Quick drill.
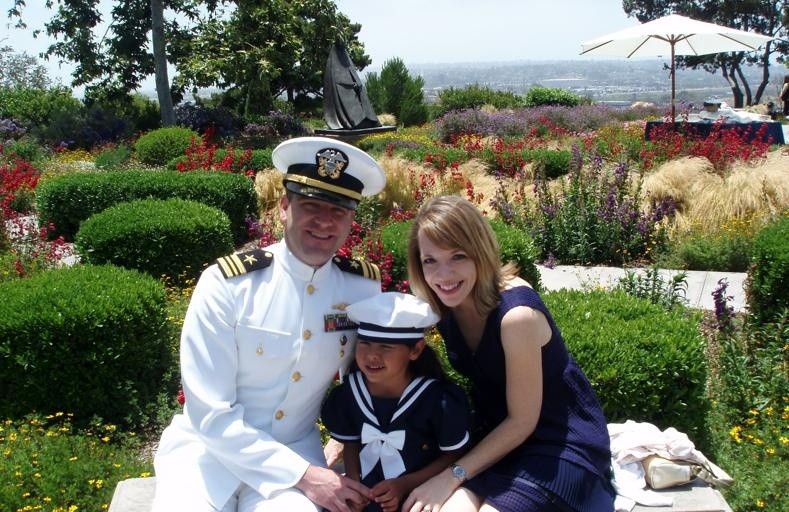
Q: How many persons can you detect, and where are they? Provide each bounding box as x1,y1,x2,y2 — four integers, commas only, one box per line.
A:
401,195,612,511
780,76,789,120
144,135,386,512
320,291,471,512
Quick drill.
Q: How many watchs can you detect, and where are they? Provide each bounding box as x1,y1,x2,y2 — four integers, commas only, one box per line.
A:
449,463,468,484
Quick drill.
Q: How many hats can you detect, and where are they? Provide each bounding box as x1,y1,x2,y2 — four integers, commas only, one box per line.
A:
344,292,440,339
271,137,386,211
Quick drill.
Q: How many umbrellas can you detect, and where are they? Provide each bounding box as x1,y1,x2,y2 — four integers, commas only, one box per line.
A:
577,13,776,132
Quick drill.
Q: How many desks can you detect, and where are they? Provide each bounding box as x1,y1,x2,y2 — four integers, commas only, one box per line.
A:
645,121,786,145
